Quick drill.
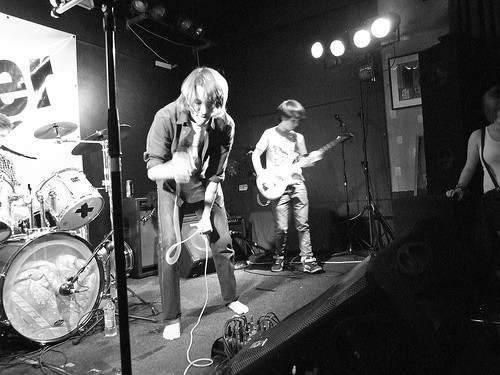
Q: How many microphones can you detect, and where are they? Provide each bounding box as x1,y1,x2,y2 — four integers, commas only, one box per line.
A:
50,0,82,19
334,114,346,128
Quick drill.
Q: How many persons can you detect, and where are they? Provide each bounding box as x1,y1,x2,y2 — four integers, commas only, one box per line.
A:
146,67,249,342
252,99,324,274
0,113,16,180
446,86,500,325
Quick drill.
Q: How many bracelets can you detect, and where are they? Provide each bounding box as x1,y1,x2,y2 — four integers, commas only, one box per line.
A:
455,185,466,191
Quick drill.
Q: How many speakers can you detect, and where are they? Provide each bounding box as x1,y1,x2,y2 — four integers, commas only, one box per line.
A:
392,198,477,312
178,220,216,279
419,38,490,196
122,197,158,278
376,214,482,325
222,253,442,375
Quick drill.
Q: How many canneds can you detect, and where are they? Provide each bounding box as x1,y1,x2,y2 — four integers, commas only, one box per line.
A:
126,180,133,197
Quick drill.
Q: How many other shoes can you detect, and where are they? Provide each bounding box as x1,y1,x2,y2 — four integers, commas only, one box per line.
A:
303,262,323,274
271,259,284,271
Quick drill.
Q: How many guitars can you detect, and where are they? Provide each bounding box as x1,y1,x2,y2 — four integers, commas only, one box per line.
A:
255,132,355,200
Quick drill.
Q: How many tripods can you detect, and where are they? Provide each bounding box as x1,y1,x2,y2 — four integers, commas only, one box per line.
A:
57,138,159,344
331,81,395,257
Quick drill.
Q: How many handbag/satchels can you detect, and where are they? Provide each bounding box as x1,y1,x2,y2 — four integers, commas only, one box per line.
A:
481,187,500,233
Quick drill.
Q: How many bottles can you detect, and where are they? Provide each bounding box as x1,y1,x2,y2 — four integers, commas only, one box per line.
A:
126,178,134,198
104,294,117,337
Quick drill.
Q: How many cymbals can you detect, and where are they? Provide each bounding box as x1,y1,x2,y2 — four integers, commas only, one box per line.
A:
33,121,77,140
71,124,132,156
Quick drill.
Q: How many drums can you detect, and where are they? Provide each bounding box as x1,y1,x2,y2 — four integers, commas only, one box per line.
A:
0,173,18,244
0,230,104,343
37,165,105,231
